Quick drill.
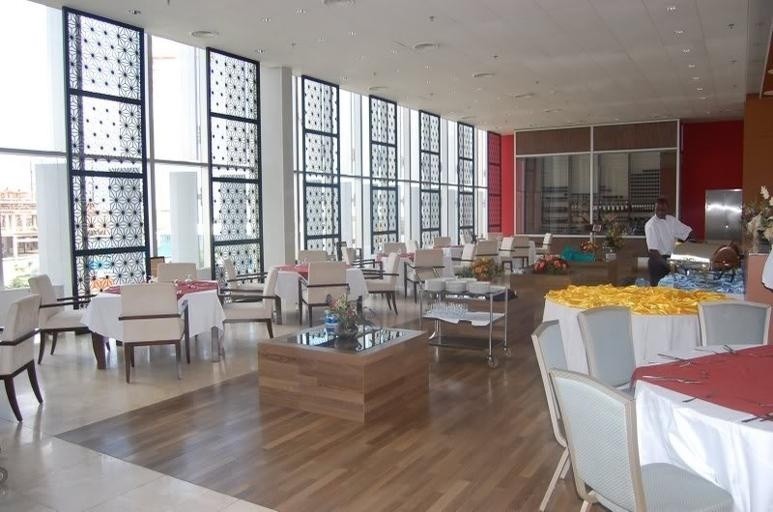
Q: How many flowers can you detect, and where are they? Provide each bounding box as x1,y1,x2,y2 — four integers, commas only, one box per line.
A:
603,226,626,252
739,187,771,253
471,256,498,280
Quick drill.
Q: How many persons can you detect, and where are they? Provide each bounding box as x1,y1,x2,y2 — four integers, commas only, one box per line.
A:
645,198,693,283
534,252,548,269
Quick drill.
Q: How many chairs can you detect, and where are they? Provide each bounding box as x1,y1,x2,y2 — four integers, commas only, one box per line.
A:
499,238,514,273
476,241,498,255
698,302,771,347
453,244,474,275
341,246,375,270
119,283,191,383
363,254,398,315
531,319,572,511
404,248,445,303
298,260,349,326
1,294,43,421
218,269,274,354
298,250,326,263
157,263,196,283
576,306,636,393
514,237,529,270
434,236,450,247
225,259,263,294
536,233,551,255
550,366,734,511
28,275,110,365
384,241,407,257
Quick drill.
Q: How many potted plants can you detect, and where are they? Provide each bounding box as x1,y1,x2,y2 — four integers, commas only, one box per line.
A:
323,293,378,337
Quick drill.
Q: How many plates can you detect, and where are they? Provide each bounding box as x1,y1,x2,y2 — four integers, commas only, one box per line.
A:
489,285,506,293
424,278,445,291
445,280,466,294
436,276,457,291
456,278,477,292
468,281,491,295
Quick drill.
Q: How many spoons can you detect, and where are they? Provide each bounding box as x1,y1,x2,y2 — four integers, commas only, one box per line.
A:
740,411,773,423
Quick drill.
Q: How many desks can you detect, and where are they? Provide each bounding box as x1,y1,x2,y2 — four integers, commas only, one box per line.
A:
277,266,369,326
544,284,730,377
632,342,772,512
80,281,226,369
